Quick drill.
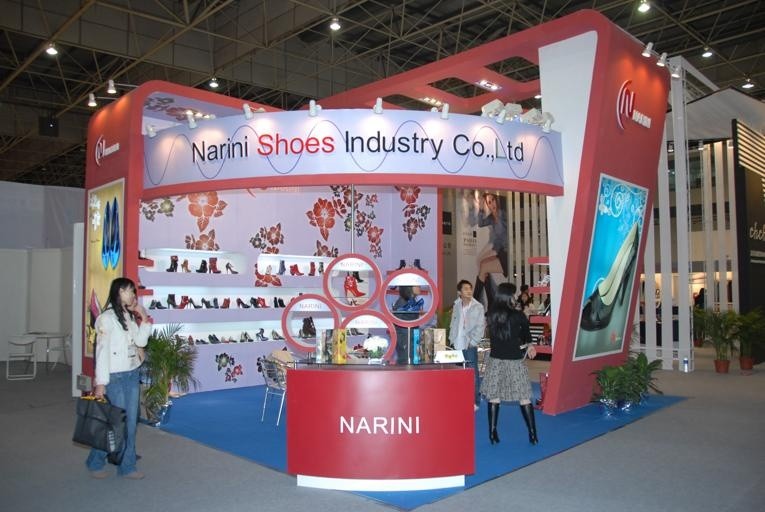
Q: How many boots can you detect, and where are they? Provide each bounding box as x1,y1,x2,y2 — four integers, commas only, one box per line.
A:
303,317,316,337
488,402,499,444
473,274,494,316
166,256,239,273
277,260,323,275
520,403,538,445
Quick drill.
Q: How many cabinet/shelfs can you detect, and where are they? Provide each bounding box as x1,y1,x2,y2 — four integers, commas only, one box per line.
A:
527,256,552,355
136,248,430,394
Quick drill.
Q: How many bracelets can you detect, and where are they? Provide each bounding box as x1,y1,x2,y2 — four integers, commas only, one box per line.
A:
93,391,104,396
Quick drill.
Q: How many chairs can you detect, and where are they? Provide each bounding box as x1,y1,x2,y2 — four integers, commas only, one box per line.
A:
259,358,293,427
5,337,37,381
50,333,73,372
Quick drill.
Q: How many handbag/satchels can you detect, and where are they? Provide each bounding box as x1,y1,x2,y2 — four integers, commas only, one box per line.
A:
72,394,128,454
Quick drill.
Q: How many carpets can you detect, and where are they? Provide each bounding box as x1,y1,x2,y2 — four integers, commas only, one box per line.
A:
157,374,690,510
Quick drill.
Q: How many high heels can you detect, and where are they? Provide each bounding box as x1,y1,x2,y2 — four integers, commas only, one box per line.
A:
102,198,120,270
188,329,283,344
397,259,423,270
149,294,285,309
581,223,638,331
344,272,365,296
396,297,424,311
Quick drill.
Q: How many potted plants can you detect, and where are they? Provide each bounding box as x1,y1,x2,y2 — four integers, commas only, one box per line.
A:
693,306,764,374
588,350,664,417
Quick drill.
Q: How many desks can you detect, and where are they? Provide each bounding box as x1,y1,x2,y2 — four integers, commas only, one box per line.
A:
24,332,70,378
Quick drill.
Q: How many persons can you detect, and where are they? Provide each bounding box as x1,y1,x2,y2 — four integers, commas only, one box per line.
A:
520,292,529,309
106,283,155,464
518,284,532,304
695,287,708,310
447,280,487,413
468,191,508,301
478,282,540,445
80,276,151,483
